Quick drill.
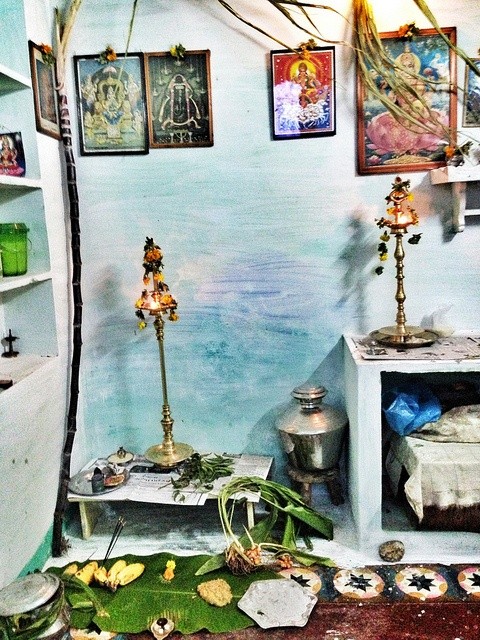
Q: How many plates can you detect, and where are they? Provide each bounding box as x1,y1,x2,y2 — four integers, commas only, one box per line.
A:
104,475,124,486
237,579,318,629
85,472,93,480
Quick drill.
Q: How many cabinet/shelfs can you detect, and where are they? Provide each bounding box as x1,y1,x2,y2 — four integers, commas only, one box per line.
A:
1,0,72,596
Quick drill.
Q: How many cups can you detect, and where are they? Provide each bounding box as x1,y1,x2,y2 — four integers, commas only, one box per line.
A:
92,474,103,493
0,222,33,277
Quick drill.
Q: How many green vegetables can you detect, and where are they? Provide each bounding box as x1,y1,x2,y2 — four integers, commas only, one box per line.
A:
170,452,334,572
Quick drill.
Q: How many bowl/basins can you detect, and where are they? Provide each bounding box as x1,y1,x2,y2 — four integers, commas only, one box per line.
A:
106,451,134,467
0,573,64,640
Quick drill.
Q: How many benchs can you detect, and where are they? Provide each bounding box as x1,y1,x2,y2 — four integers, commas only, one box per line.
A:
67,456,273,539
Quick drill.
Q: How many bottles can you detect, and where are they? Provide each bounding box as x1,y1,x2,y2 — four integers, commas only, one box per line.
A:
274,384,347,472
117,447,126,458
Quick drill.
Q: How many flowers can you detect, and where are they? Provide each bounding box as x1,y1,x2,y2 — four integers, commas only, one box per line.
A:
300,38,318,58
40,44,57,66
169,43,186,58
96,45,116,63
397,20,420,41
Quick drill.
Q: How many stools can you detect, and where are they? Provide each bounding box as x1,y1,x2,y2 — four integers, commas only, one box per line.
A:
285,465,344,506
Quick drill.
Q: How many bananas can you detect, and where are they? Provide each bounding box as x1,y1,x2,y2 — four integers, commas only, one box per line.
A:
64,561,145,592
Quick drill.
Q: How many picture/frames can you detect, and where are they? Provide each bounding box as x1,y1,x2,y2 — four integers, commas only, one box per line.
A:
28,40,63,141
270,46,336,140
73,51,149,156
144,50,213,148
461,58,480,128
355,27,457,176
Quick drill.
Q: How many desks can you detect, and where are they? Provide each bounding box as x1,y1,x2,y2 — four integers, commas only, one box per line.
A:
341,334,480,554
